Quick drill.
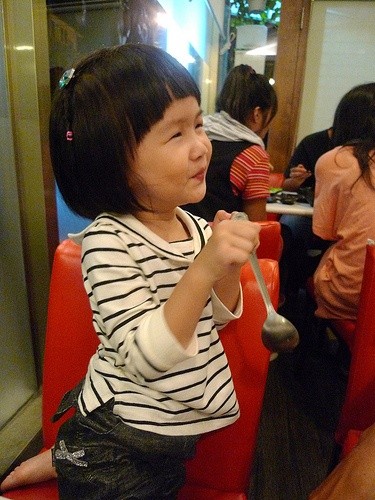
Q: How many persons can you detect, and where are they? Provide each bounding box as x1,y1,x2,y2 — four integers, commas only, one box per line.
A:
278,83,375,378
181,65,300,309
0,43,260,500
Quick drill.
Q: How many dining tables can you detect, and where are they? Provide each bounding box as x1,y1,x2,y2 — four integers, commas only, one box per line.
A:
266,200,314,215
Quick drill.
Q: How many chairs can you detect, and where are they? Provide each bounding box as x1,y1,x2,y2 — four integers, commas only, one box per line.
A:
307,238,375,445
207,221,284,263
1,239,279,500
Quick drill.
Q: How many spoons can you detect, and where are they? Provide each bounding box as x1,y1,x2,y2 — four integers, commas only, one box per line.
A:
231,212,299,354
